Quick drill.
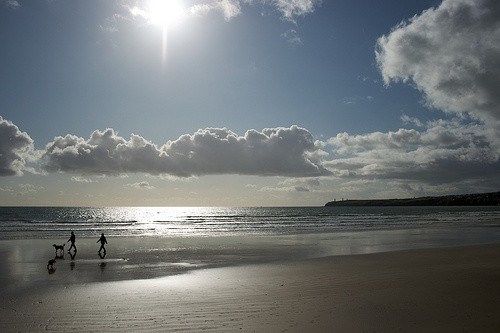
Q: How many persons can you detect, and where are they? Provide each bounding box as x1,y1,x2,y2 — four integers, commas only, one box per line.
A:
66,231,78,252
95,233,108,253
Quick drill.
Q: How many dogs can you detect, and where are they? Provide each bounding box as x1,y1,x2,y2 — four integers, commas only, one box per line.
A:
47,259,56,265
52,243,65,252
